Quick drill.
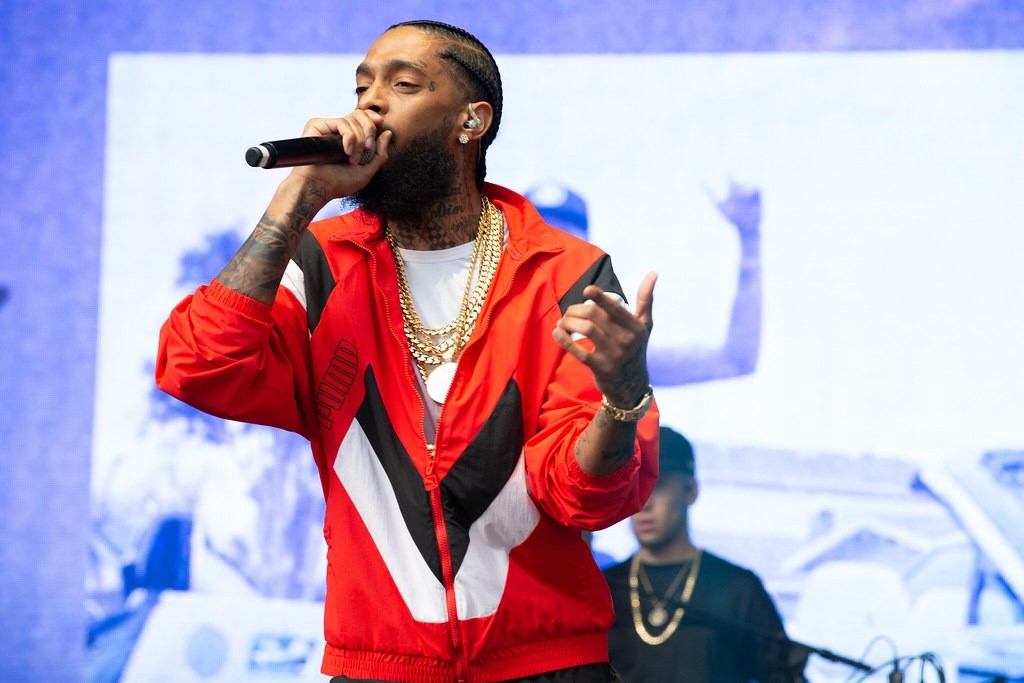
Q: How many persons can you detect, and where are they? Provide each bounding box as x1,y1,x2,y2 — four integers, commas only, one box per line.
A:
153,17,664,683
521,156,765,392
95,231,323,681
589,425,800,683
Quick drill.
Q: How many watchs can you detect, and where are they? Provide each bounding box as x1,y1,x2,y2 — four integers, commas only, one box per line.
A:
595,379,654,422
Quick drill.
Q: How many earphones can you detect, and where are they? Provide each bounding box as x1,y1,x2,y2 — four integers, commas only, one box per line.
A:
464,110,482,129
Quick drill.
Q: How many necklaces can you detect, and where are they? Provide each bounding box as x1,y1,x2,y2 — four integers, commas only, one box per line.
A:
630,543,703,643
384,187,509,396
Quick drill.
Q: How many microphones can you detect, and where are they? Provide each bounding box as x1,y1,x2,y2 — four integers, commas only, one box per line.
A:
245,133,377,170
